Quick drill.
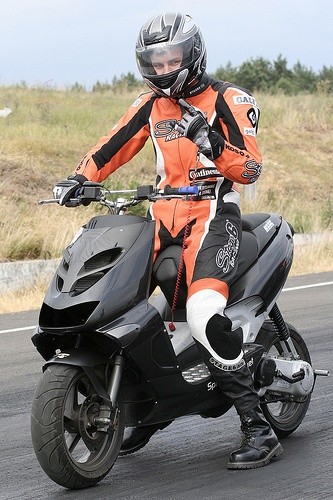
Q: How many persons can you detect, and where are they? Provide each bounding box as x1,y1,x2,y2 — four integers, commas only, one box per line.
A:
53,9,283,468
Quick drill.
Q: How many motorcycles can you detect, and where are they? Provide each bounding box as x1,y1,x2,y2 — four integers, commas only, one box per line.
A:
30,178,330,489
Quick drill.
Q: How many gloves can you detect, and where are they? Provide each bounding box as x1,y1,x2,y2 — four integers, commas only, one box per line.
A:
176,98,210,146
52,173,92,206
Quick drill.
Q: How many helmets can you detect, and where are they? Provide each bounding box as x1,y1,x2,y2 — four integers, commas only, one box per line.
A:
137,11,207,95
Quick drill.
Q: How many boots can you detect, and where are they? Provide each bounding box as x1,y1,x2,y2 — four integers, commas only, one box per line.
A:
119,421,175,455
228,404,284,468
